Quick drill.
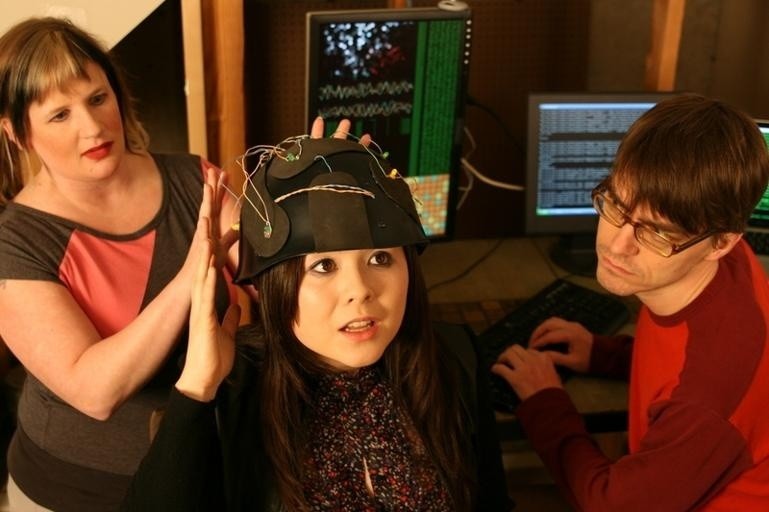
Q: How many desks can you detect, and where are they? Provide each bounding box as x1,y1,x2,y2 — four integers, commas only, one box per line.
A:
237,237,767,443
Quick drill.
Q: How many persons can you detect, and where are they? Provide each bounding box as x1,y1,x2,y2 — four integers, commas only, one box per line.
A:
115,137,514,512
2,16,373,512
491,92,769,511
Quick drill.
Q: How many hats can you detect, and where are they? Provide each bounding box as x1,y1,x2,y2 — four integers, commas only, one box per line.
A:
232,139,427,286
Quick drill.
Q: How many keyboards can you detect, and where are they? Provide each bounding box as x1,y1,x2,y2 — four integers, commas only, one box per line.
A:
483,279,629,415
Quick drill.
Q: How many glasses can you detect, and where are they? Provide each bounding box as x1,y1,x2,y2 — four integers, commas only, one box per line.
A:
590,177,722,256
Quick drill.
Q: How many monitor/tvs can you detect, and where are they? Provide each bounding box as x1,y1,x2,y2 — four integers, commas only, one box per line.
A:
526,92,679,234
304,9,472,244
747,117,769,233
